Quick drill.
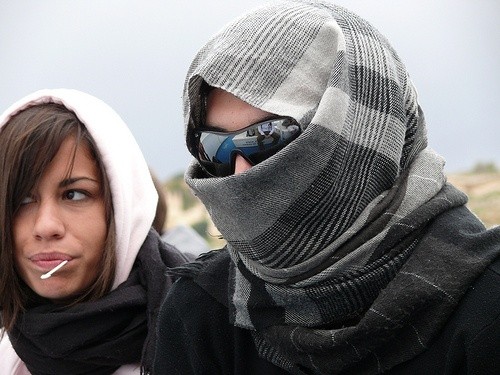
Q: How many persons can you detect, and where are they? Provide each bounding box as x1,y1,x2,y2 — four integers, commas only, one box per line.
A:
0,88,198,375
138,1,500,375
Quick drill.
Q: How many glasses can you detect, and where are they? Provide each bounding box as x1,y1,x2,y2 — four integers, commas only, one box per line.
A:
190,110,308,179
